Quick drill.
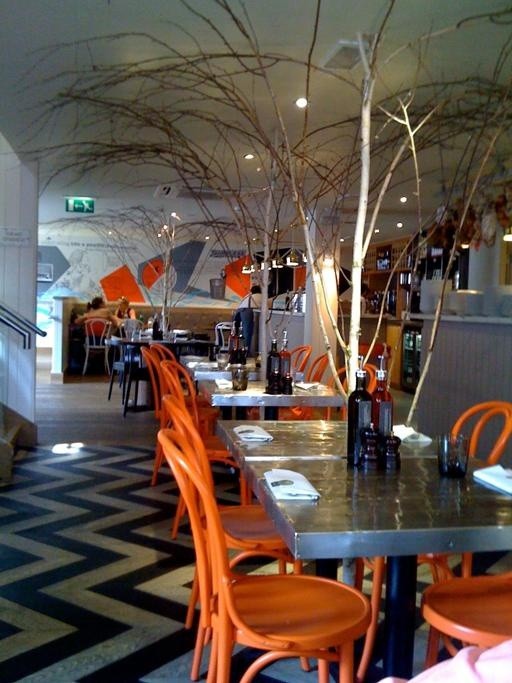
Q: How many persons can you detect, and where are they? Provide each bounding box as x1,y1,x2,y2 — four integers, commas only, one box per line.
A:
114,296,136,324
361,283,368,316
74,297,117,346
230,285,262,352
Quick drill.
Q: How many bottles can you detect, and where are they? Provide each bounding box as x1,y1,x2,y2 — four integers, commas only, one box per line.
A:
347,356,401,469
153,314,159,340
123,310,128,320
266,329,293,394
376,248,389,270
370,290,396,316
139,311,144,322
294,293,299,313
255,352,262,367
159,329,163,340
285,290,290,311
229,321,247,364
301,292,306,313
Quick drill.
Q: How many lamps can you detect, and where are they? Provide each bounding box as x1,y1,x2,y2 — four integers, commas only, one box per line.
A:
241,213,308,275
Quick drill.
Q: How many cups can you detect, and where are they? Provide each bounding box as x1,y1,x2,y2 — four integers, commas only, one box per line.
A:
187,331,194,341
216,353,230,369
132,330,141,339
209,345,220,362
437,433,470,478
168,332,176,343
232,367,249,391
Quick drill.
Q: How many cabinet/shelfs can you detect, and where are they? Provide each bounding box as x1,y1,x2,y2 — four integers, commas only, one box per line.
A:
362,234,425,388
426,236,469,291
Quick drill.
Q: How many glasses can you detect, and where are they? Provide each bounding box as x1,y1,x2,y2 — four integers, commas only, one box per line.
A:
119,296,128,303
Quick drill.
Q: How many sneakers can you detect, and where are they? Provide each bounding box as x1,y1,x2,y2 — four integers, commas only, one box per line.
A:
232,322,243,337
